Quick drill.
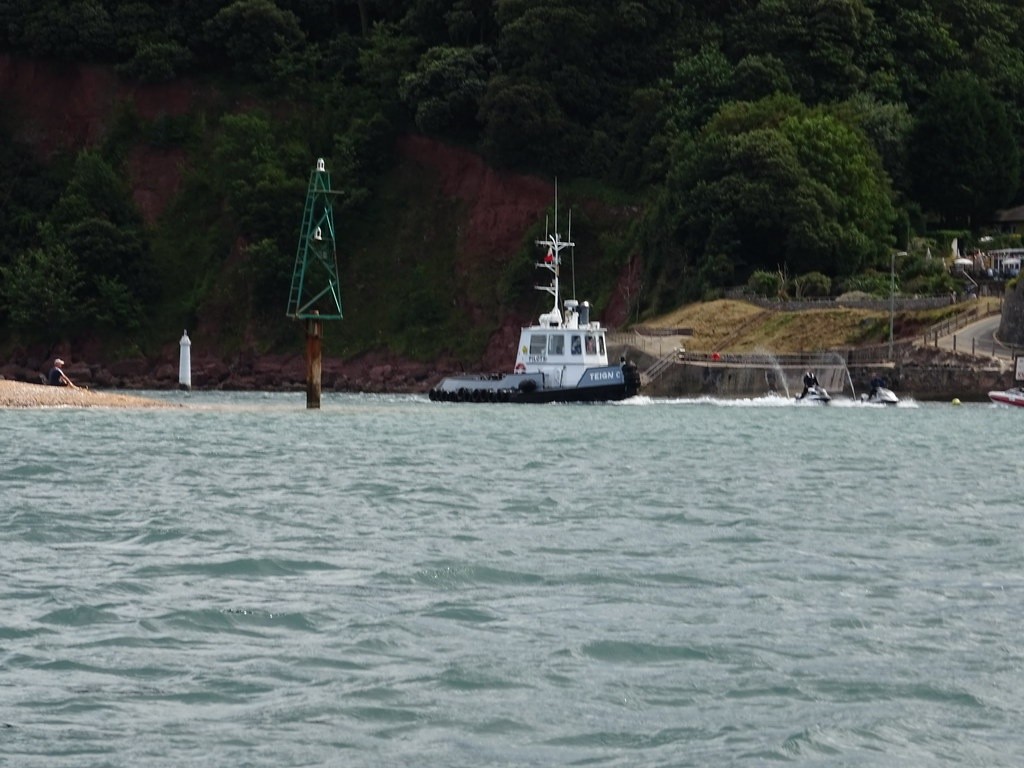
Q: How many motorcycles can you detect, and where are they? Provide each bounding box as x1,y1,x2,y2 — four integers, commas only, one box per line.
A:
861,387,899,405
796,386,831,404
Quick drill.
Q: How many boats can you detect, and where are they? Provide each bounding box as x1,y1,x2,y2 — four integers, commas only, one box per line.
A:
430,175,639,402
987,387,1024,407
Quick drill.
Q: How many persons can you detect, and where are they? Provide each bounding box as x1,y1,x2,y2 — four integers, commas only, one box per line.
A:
794,369,819,399
867,373,884,401
46,358,88,390
571,336,592,354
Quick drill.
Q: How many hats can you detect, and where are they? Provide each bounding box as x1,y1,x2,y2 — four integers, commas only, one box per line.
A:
55,359,64,365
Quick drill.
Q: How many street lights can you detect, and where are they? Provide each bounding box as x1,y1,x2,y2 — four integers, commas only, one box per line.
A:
889,252,909,362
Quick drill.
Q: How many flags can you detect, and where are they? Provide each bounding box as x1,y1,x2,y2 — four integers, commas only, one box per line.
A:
544,246,553,261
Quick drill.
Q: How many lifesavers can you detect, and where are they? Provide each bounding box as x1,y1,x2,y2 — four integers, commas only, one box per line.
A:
515,363,526,374
711,352,720,362
428,386,518,403
